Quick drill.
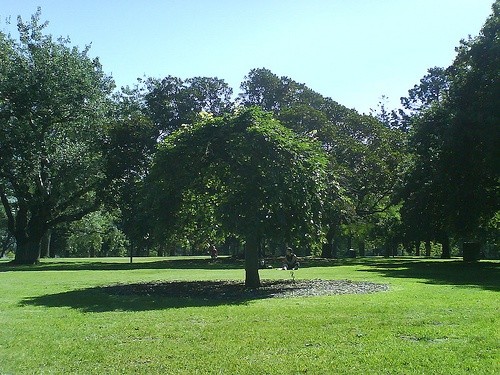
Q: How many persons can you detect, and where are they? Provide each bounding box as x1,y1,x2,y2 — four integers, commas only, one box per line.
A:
282,247,300,270
210,245,218,264
